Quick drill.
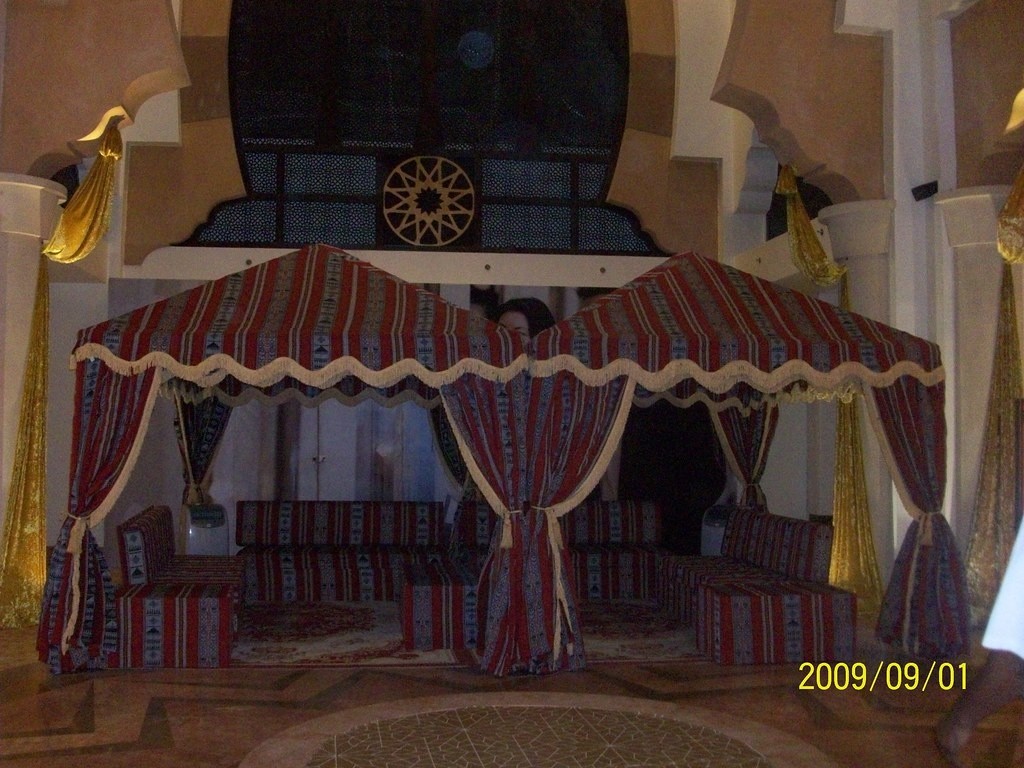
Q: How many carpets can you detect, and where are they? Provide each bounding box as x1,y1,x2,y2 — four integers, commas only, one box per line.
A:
233,599,712,667
237,692,841,768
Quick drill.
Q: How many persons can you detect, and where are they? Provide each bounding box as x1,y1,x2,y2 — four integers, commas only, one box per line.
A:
495,300,556,342
934,520,1024,768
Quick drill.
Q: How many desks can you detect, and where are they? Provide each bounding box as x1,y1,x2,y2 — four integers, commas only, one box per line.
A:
396,565,478,650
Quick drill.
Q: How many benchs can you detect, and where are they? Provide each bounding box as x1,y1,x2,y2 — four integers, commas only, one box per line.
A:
234,502,665,603
692,580,859,665
661,508,832,630
112,503,239,669
